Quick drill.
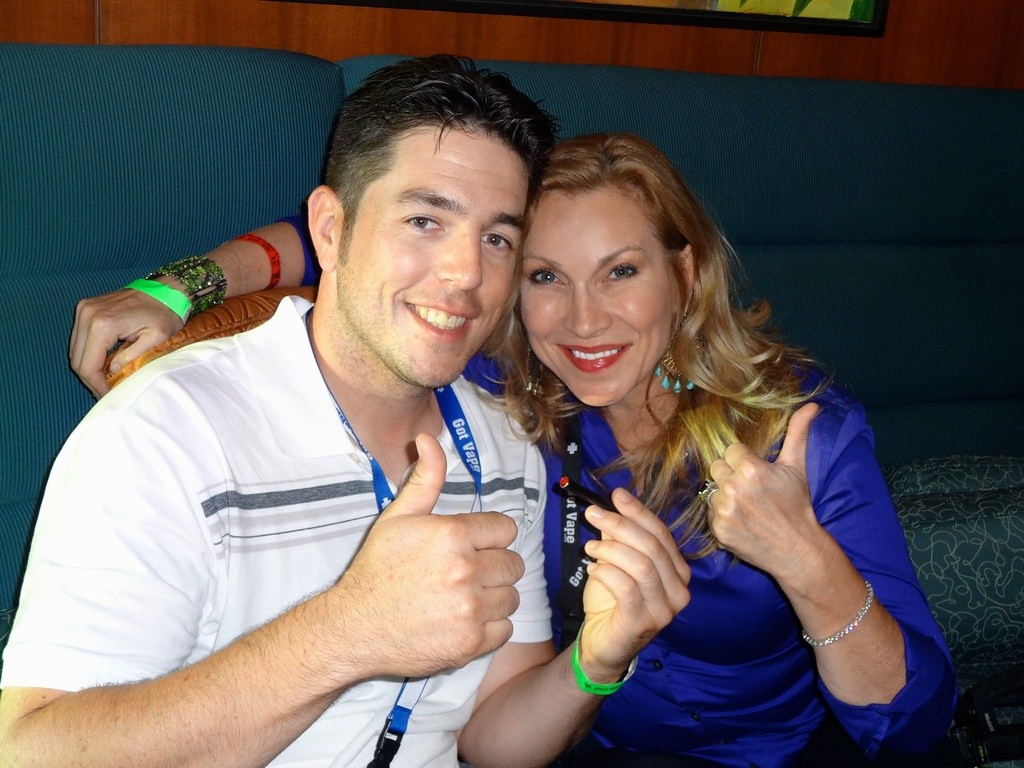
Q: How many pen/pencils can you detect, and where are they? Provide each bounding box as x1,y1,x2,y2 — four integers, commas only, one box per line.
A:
559,477,675,539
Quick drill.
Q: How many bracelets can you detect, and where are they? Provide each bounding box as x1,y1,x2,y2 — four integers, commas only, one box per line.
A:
572,620,640,695
144,255,228,318
221,235,282,291
802,578,874,647
121,279,192,325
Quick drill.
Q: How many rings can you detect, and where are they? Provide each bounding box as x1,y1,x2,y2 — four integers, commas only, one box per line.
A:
698,478,719,509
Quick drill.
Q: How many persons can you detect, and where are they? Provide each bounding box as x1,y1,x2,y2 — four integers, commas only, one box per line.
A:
68,132,957,768
1,56,690,768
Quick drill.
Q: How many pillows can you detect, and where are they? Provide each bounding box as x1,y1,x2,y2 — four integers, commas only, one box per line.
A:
882,456,1024,696
104,285,316,390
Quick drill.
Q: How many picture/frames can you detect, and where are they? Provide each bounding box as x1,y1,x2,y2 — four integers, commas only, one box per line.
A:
278,0,895,41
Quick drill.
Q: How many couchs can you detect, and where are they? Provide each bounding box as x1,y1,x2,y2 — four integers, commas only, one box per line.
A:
1,37,1024,768
